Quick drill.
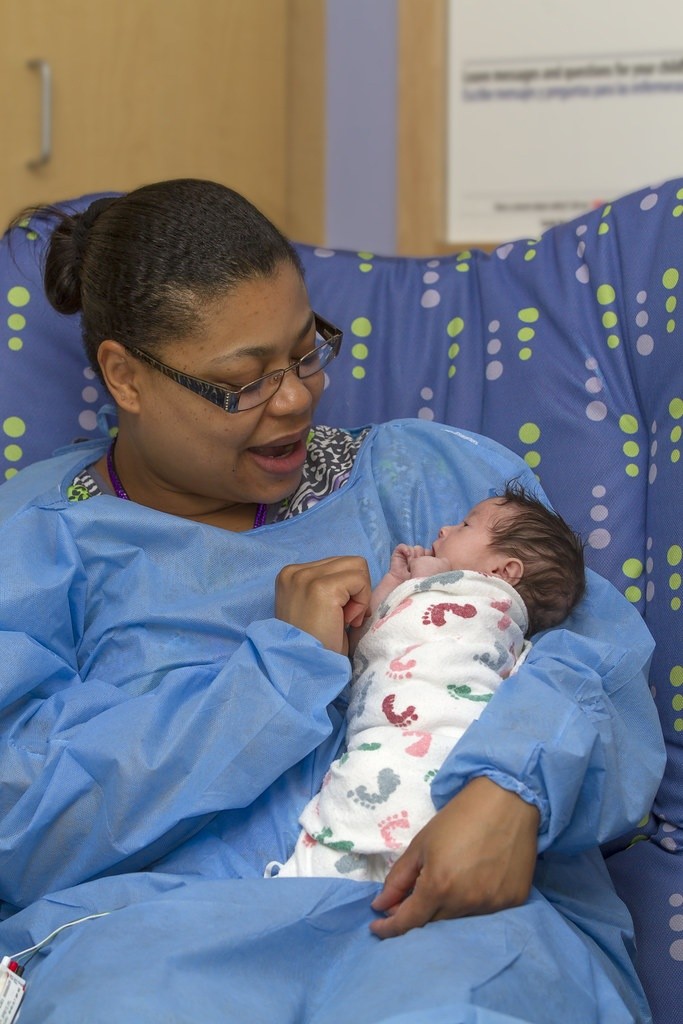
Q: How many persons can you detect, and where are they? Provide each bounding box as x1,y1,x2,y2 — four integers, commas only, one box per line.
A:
252,478,588,881
0,173,667,1024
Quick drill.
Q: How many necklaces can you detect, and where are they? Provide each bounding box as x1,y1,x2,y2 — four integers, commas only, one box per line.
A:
101,434,268,531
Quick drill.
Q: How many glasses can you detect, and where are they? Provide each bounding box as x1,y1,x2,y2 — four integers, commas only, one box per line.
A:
92,310,344,415
